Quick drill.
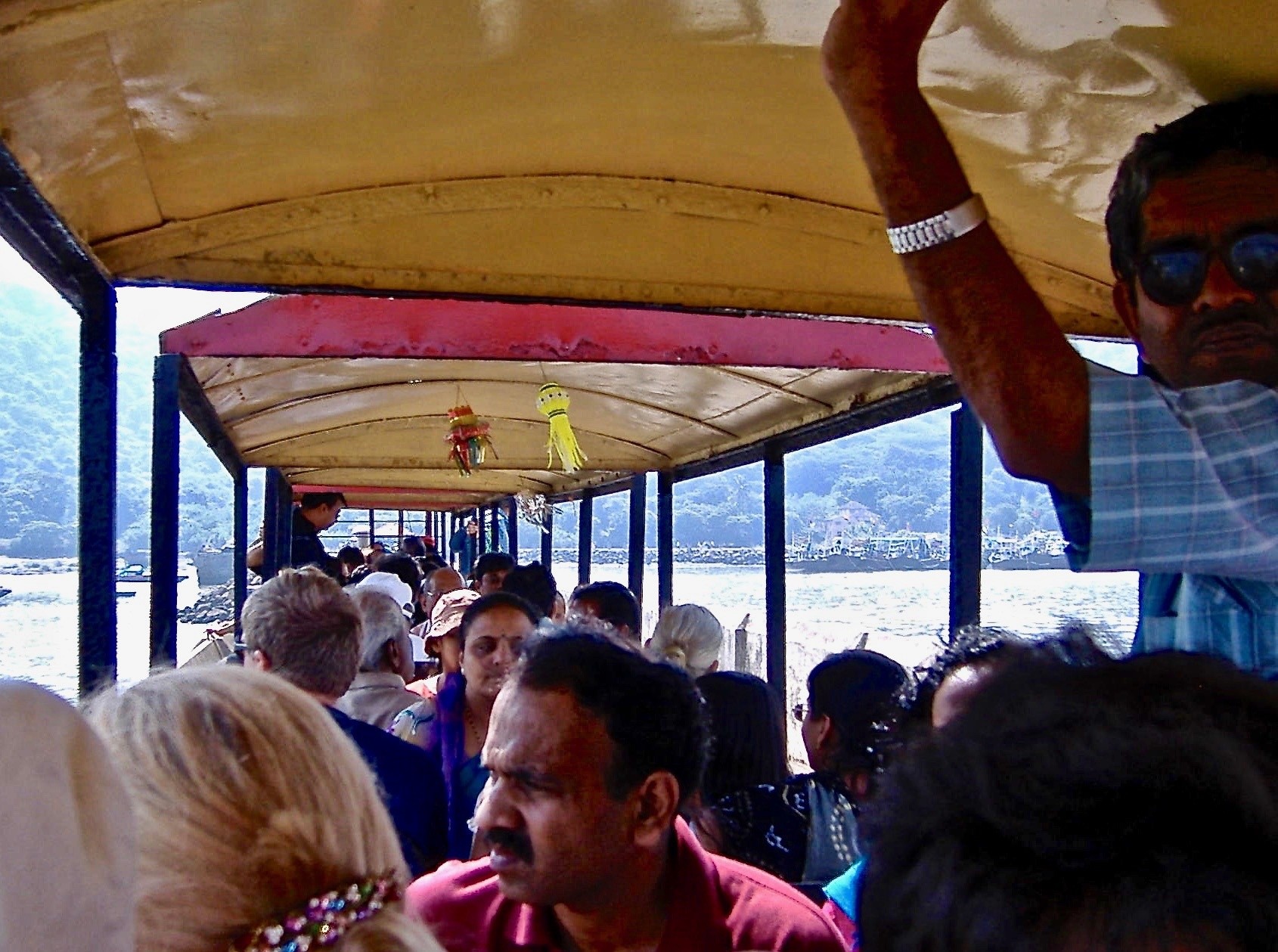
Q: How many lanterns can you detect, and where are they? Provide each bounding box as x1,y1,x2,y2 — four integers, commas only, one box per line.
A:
442,405,500,481
539,385,586,473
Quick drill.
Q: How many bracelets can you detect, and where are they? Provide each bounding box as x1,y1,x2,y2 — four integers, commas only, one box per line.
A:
884,195,985,257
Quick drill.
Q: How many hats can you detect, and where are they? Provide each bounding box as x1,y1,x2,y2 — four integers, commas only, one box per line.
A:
423,537,435,545
353,571,415,618
425,589,482,657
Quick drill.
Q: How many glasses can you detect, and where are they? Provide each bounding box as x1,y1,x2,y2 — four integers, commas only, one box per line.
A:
1119,229,1278,306
791,704,822,721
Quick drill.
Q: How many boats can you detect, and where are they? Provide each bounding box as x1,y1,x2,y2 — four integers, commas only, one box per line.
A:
114,564,189,584
116,591,137,597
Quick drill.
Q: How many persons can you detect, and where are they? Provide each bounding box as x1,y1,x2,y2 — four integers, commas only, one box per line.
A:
268,491,344,579
0,520,1278,952
819,0,1278,679
